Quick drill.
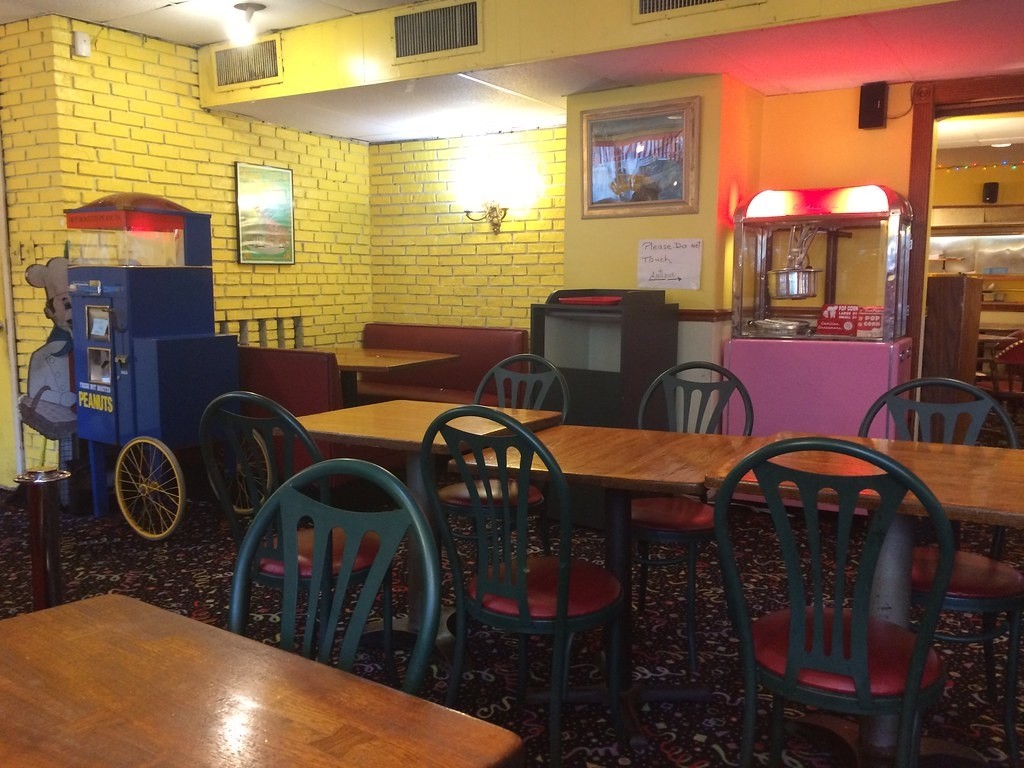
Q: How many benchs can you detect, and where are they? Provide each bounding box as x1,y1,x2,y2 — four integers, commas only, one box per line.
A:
237,345,405,487
358,321,528,407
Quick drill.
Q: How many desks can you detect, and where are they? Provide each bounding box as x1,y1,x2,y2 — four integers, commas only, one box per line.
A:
705,432,1023,768
290,345,461,407
268,400,564,689
0,593,520,768
446,424,755,746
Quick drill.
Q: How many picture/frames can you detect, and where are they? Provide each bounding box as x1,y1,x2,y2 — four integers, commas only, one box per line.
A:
234,161,296,265
579,95,700,218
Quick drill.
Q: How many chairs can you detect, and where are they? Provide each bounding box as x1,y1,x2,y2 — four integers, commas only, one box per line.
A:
199,352,1024,768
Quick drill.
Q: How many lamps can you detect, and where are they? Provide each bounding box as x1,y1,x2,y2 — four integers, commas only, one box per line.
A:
448,170,540,237
